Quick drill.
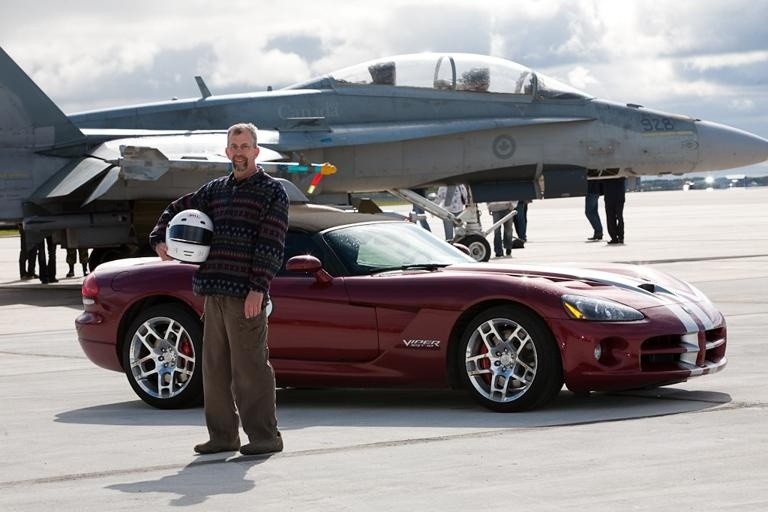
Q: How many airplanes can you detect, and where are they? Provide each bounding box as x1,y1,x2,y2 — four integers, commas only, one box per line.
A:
0,46,767,276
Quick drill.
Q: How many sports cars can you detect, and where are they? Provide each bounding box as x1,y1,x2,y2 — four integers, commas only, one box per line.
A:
76,205,731,414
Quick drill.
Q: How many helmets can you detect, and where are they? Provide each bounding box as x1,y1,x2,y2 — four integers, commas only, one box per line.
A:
165,209,214,262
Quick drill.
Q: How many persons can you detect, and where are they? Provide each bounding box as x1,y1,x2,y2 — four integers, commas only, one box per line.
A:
35,229,59,285
601,168,625,244
585,190,604,240
486,198,515,258
64,236,89,277
410,186,431,232
149,120,290,461
435,182,471,244
18,223,38,279
511,195,531,240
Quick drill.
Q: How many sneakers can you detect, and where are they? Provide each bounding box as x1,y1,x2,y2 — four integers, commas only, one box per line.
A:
241,442,283,455
196,440,239,454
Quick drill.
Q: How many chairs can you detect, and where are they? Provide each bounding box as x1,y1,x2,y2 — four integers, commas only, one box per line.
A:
330,234,379,275
